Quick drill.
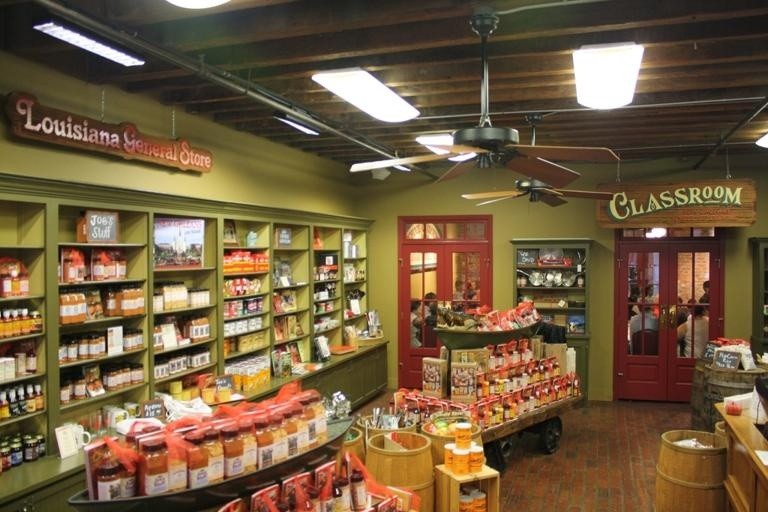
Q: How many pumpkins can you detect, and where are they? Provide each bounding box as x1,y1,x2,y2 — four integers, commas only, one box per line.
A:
726,401,741,415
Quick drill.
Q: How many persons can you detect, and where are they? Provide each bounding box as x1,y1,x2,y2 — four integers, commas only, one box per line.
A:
410,279,480,348
630,281,709,358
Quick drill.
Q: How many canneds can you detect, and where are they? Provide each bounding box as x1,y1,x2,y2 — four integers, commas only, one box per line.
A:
172,385,201,401
153,313,210,351
223,294,263,318
60,382,70,404
0,432,46,472
59,328,144,365
217,387,231,402
201,388,216,403
66,361,143,401
125,390,327,497
167,381,182,393
443,422,485,475
459,486,487,512
95,462,123,500
154,346,211,379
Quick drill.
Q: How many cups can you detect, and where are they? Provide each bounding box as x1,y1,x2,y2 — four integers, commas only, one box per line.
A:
72,425,91,450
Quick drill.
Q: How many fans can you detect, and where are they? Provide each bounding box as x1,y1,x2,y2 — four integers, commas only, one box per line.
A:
461,116,622,210
348,6,622,189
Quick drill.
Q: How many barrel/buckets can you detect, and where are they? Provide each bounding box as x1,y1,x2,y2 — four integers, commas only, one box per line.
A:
325,407,486,512
652,358,768,511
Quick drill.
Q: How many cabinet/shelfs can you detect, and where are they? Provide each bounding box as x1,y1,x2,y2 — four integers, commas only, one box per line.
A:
0,172,389,511
510,238,594,407
